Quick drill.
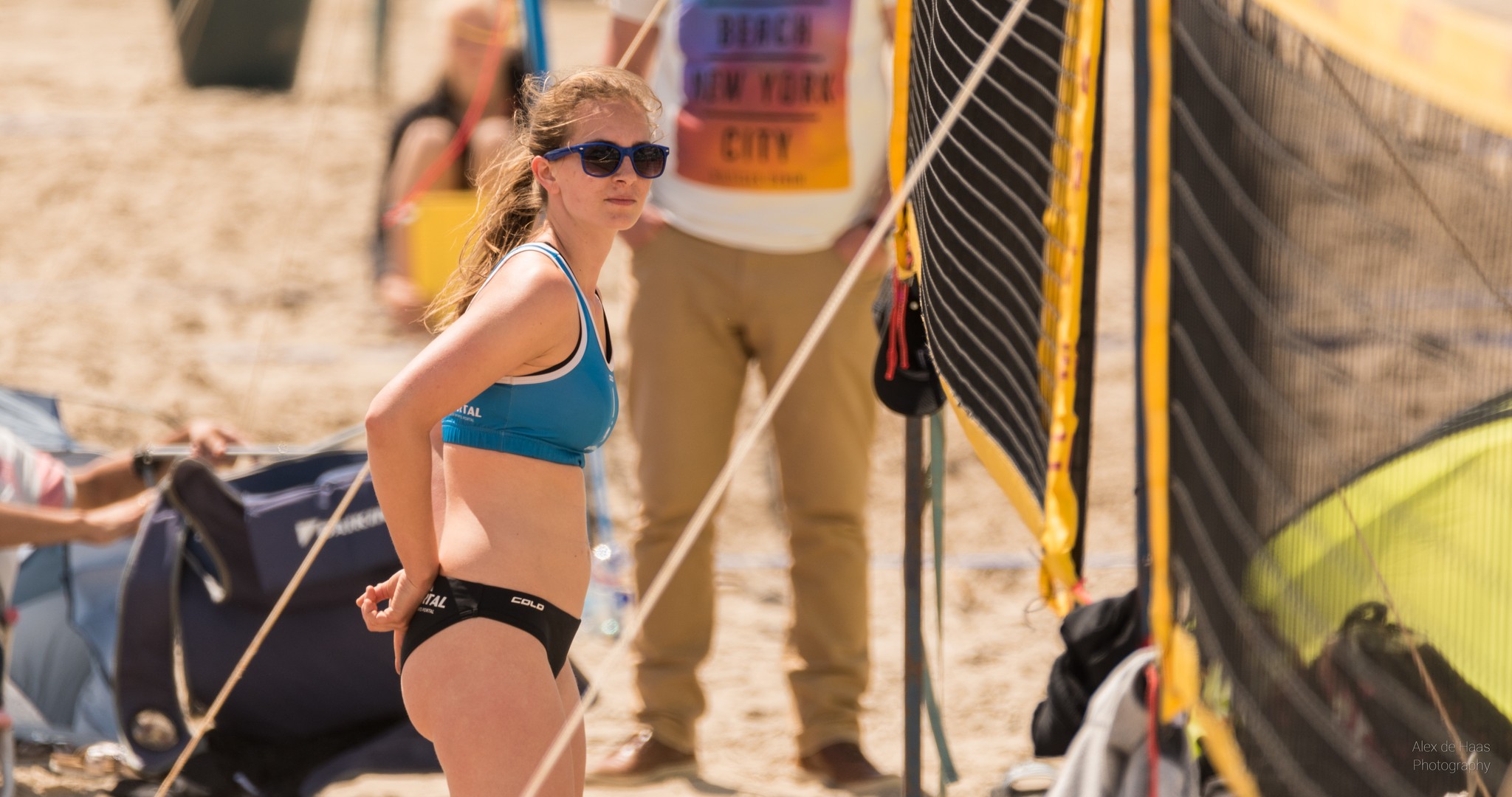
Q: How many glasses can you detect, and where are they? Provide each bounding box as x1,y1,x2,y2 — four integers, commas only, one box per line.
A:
542,142,669,179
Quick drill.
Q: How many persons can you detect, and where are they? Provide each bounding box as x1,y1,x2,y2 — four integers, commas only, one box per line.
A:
587,0,895,789
0,421,259,739
355,67,670,797
375,2,542,322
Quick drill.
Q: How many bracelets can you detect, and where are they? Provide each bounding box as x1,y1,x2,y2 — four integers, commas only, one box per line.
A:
130,447,161,494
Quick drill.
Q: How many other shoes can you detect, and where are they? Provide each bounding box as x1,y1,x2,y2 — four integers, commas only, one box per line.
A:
795,742,887,787
586,738,695,785
